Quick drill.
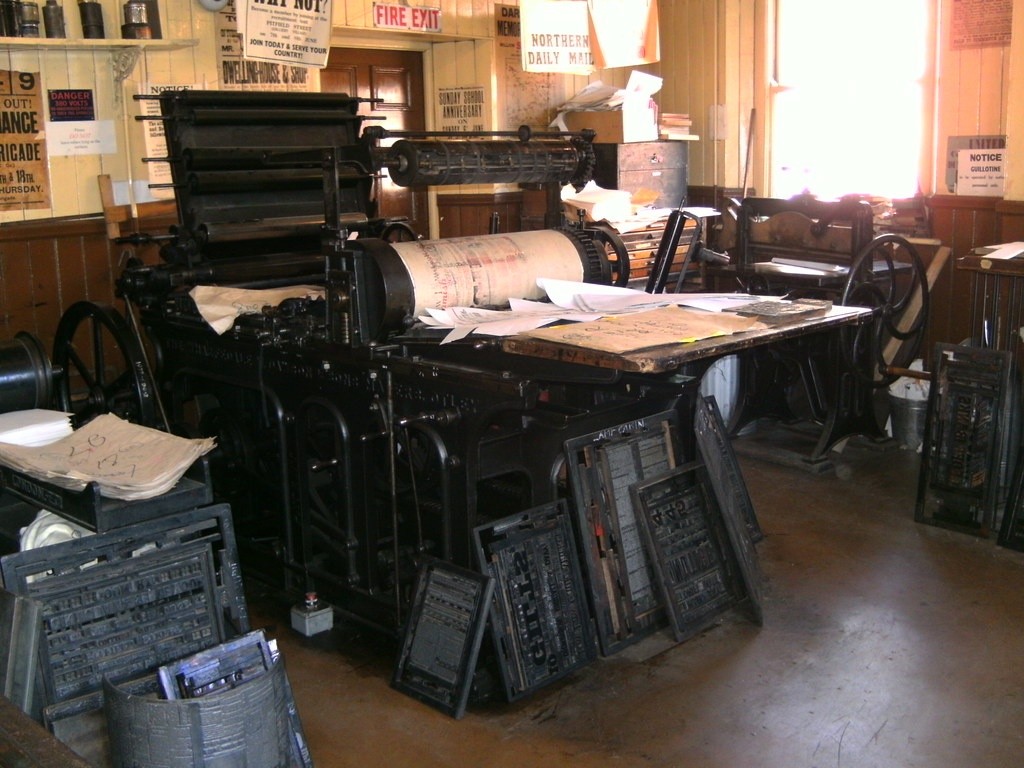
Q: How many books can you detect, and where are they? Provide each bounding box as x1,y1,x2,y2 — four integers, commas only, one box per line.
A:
721,300,825,326
658,113,701,141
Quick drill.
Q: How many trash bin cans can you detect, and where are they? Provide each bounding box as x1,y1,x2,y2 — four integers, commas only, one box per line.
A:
886,391,928,451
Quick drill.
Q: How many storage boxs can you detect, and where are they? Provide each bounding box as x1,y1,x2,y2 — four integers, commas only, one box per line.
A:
524,109,706,293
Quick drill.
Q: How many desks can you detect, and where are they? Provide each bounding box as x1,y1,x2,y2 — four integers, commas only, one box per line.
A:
502,293,873,372
709,259,912,463
